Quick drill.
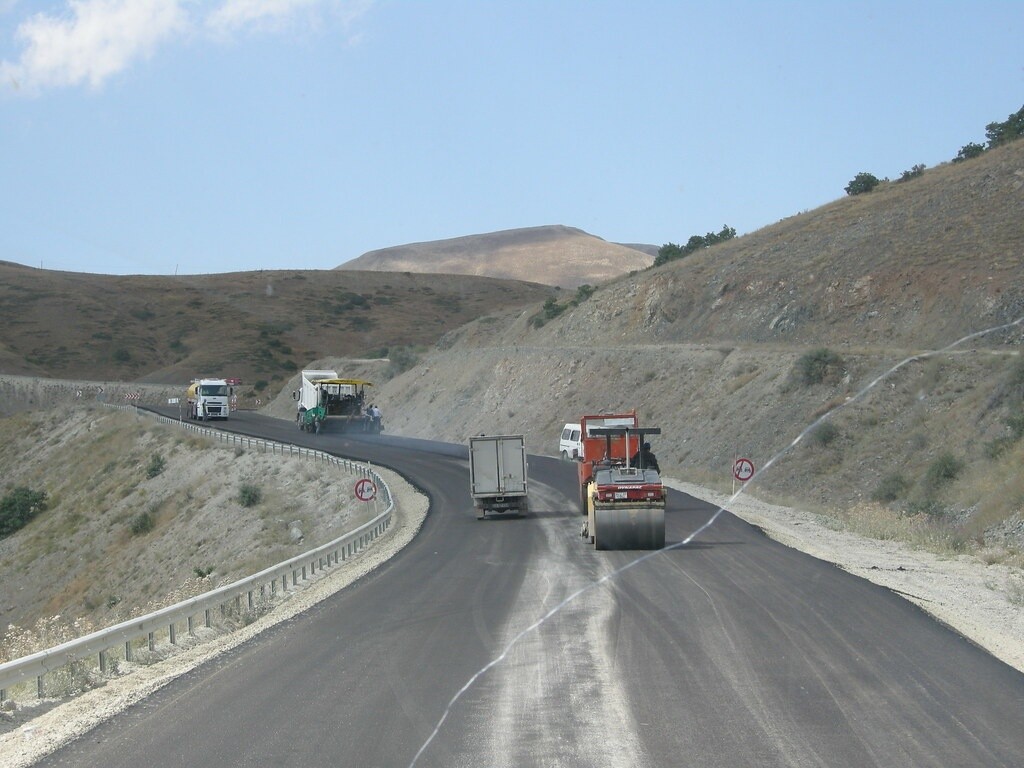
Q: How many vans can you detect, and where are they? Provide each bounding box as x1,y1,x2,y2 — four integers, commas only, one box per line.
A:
558,422,607,462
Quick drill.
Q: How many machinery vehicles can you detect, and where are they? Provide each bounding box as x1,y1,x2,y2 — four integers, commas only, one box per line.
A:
293,369,375,436
577,409,668,551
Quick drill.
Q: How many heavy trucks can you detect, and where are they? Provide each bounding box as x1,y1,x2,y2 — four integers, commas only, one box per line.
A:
185,377,233,422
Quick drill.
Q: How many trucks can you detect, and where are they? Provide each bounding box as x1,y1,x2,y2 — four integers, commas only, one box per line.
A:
468,433,529,520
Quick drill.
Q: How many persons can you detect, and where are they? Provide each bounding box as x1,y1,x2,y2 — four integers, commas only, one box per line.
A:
364,404,383,436
630,442,660,475
